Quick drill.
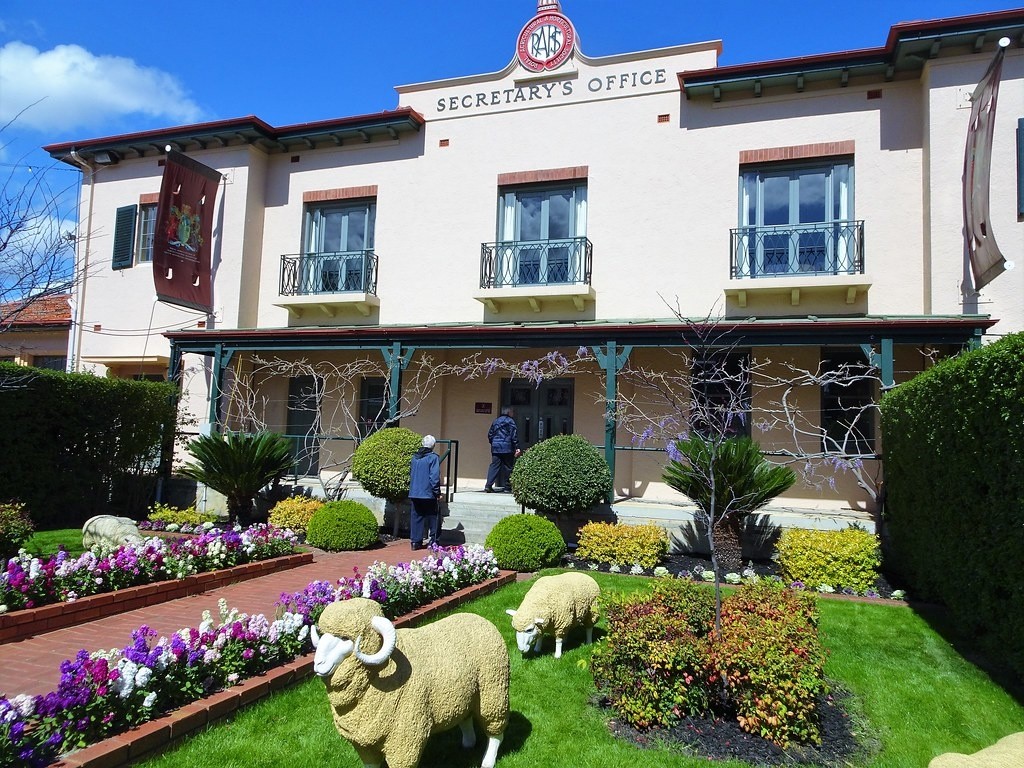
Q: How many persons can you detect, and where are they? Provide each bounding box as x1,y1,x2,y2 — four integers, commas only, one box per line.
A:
484,404,522,493
409,435,442,551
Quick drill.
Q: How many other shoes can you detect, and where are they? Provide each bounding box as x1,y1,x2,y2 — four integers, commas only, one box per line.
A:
486,487,495,493
504,490,512,494
411,542,421,551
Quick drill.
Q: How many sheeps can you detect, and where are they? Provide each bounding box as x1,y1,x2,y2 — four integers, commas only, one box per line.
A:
505,572,600,658
311,597,510,768
